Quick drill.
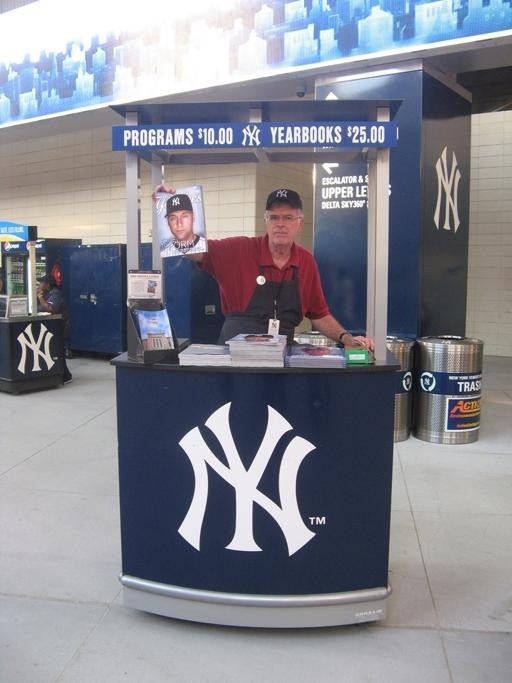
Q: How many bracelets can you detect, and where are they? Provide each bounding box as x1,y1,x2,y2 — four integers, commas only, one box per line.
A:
339,331,351,340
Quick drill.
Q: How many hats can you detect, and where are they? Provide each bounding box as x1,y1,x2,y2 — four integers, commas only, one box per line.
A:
165,195,191,216
37,275,56,286
266,190,301,210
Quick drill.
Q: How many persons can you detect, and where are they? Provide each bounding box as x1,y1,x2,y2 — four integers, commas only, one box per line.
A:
152,183,375,351
37,274,70,343
158,191,204,257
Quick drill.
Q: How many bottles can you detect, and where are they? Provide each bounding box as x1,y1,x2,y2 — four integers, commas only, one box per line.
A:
9,256,45,295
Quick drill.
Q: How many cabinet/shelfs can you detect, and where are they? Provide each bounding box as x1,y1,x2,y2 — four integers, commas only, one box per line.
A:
65,244,126,358
138,242,223,342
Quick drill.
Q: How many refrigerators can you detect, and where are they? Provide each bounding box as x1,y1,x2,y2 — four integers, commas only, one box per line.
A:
1,237,82,299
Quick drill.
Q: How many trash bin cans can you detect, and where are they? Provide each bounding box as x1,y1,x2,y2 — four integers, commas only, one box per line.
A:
413,334,484,445
385,336,415,442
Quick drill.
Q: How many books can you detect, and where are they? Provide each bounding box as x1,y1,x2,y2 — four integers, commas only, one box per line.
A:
179,333,346,371
